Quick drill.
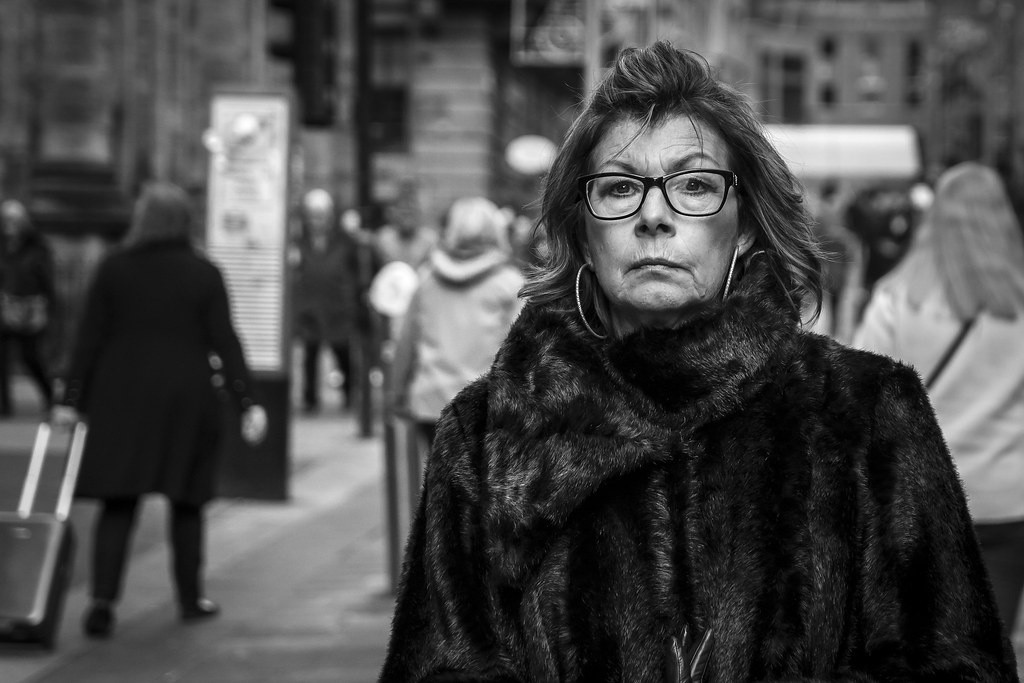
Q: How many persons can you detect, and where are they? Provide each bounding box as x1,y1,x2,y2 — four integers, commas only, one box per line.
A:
374,43,1019,683
53,182,270,636
0,145,1018,446
854,161,1022,639
378,192,533,468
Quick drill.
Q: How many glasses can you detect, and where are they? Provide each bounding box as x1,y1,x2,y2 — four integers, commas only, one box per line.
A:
575,168,741,220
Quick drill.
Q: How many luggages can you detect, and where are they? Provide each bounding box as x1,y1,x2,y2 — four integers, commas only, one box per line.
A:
0,405,93,649
